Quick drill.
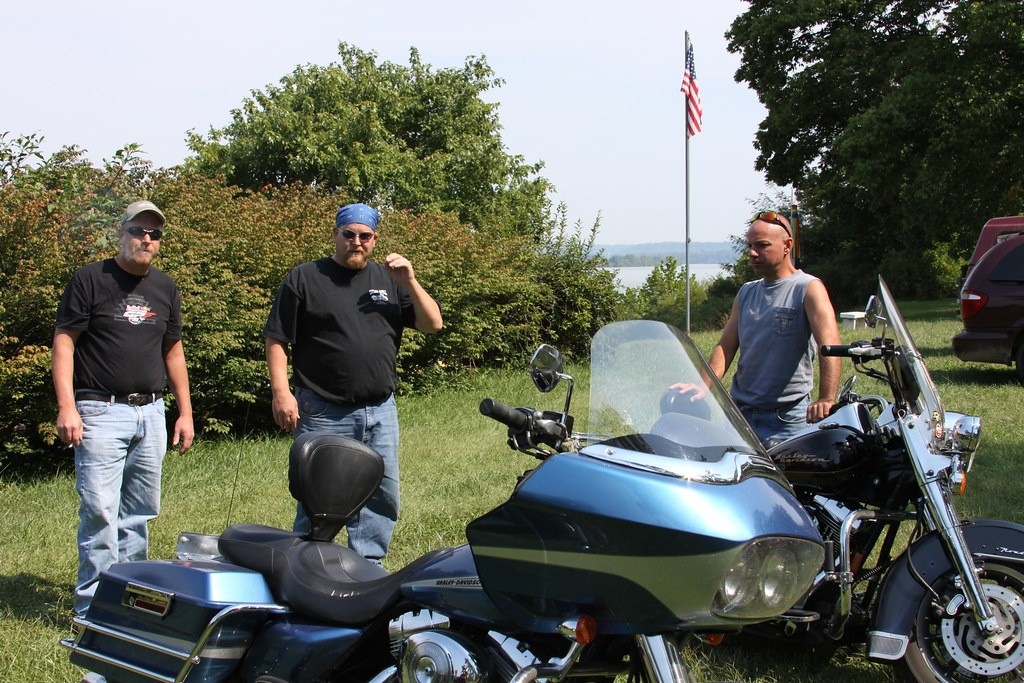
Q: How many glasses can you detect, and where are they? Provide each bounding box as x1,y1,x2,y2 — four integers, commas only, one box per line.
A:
123,226,162,240
750,211,792,237
337,228,373,242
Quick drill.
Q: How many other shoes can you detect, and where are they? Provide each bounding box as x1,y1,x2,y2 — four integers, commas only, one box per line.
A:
64,609,81,635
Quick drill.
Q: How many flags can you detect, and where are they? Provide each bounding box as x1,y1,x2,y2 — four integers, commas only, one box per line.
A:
679,36,703,139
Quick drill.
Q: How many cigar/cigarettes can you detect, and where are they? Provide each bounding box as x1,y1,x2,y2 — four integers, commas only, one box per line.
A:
67,442,74,448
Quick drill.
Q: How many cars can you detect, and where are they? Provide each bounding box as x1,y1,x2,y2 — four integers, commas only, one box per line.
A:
951,232,1024,387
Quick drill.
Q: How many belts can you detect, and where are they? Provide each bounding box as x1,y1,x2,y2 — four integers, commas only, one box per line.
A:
75,390,163,406
294,370,308,388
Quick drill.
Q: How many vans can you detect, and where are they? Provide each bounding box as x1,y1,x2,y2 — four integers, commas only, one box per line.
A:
955,213,1024,322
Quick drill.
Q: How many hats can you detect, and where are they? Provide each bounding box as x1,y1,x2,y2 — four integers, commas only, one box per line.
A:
121,200,165,225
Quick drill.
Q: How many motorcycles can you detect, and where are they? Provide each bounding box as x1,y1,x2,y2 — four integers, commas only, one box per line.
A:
57,319,829,683
645,274,1024,683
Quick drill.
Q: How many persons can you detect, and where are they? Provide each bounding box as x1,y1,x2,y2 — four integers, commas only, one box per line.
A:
667,211,842,449
263,204,442,569
52,201,196,636
